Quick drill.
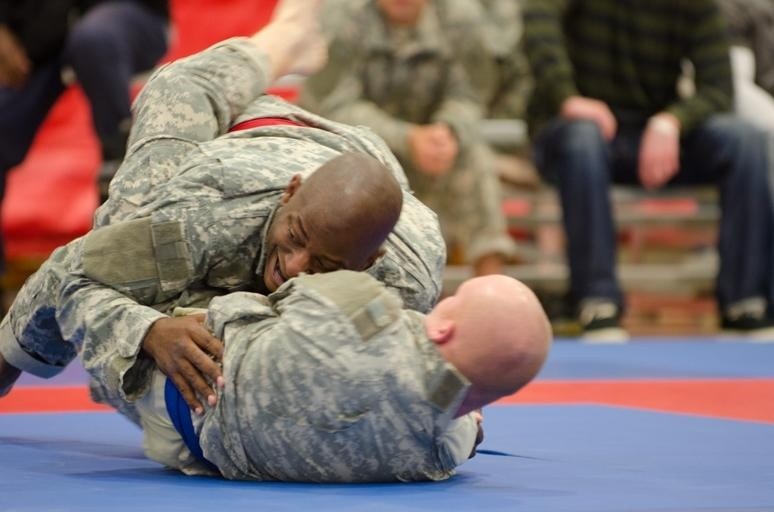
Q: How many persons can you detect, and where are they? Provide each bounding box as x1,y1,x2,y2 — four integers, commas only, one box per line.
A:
292,0,520,282
52,0,554,483
0,91,451,396
0,1,173,214
515,2,772,347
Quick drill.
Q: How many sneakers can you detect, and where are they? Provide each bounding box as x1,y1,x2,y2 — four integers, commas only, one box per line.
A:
578,297,629,345
718,297,774,342
